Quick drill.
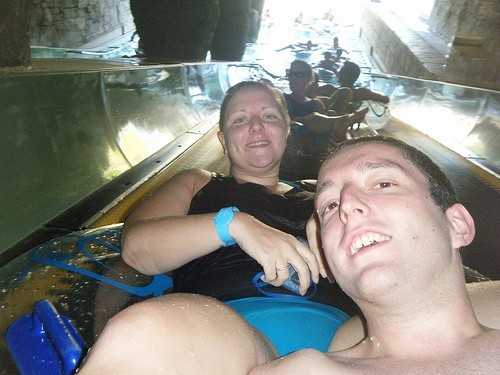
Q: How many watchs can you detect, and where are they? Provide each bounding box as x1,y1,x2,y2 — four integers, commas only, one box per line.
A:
214,206,241,246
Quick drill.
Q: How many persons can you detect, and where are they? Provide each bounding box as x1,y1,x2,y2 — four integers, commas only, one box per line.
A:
247,136,500,375
74,82,500,375
259,8,341,34
276,36,390,142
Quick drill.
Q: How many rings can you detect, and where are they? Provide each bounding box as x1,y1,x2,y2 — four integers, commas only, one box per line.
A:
276,265,288,271
